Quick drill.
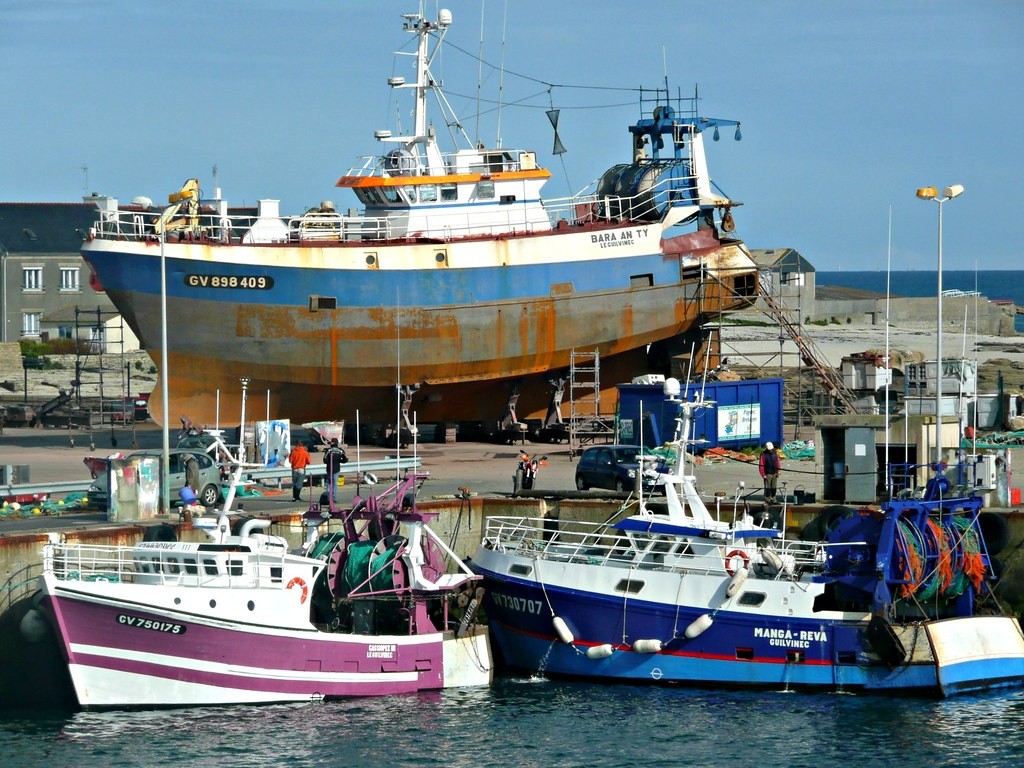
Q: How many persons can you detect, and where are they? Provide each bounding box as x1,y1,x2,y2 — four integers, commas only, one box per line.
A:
323,438,348,503
289,441,311,502
184,453,199,503
759,441,780,504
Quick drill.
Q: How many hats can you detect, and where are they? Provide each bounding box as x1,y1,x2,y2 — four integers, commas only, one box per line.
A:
766,442,774,450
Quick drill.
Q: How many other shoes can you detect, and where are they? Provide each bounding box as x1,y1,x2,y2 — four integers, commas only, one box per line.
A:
292,496,302,501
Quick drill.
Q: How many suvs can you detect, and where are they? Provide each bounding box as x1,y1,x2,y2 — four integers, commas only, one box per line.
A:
575,443,672,498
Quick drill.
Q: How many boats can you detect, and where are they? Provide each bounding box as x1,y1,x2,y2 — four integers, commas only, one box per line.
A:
0,389,496,707
461,332,1024,703
77,1,763,430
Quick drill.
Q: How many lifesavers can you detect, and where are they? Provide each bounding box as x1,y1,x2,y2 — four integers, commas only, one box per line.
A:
286,576,308,604
724,549,749,577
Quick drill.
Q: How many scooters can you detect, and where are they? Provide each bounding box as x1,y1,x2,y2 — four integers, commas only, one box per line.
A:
517,449,547,490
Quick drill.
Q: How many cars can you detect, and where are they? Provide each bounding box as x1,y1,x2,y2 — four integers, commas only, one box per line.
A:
24,358,44,370
87,448,222,511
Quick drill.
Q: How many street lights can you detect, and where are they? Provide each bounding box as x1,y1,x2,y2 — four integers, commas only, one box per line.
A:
916,185,964,486
238,375,251,464
127,189,196,513
